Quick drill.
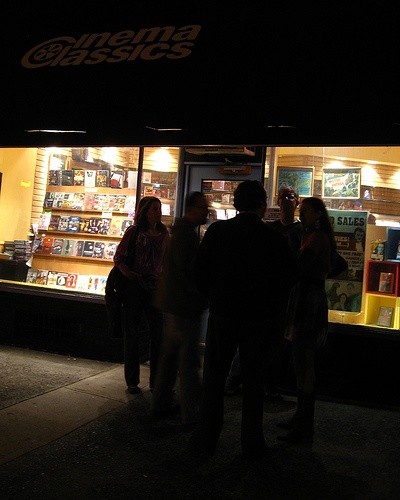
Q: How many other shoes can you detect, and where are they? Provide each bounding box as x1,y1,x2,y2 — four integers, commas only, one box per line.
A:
276,420,295,430
128,385,137,394
277,433,310,445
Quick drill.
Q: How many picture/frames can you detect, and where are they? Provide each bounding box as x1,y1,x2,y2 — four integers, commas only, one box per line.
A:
275,166,313,198
322,168,361,199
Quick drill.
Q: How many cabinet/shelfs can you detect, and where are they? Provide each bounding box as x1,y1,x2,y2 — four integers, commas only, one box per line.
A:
327,225,400,330
26,185,176,296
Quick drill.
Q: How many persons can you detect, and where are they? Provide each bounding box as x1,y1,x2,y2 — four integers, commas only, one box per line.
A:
115,181,336,458
324,226,366,311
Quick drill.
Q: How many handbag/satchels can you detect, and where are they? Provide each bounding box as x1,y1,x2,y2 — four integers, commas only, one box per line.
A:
105,268,152,325
